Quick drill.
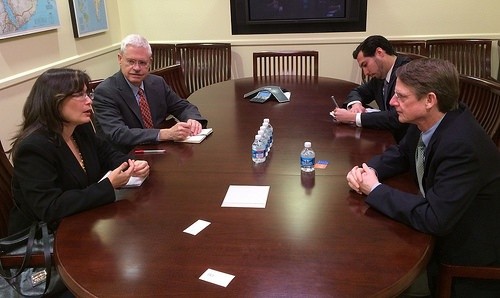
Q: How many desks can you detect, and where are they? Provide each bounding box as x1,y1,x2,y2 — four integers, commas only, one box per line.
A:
53,75,435,298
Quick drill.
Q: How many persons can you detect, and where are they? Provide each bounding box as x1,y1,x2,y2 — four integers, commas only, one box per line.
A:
4,68,149,238
346,57,500,298
334,35,422,133
92,35,208,147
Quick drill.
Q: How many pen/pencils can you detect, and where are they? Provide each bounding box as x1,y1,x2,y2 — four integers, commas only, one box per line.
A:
331,96,340,107
135,149,166,152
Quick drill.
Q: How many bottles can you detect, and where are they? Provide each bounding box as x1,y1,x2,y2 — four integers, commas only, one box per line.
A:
251,118,274,163
299,141,316,172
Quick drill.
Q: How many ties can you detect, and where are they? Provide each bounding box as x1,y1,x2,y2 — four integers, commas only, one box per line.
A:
137,89,153,129
415,135,426,199
384,80,389,112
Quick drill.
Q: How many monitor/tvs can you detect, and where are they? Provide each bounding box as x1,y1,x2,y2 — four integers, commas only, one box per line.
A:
247,0,350,23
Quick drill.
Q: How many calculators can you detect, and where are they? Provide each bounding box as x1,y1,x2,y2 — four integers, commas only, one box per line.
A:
249,91,271,103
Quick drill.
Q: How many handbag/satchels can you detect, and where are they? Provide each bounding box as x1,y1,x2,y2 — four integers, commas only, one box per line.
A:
0,221,67,298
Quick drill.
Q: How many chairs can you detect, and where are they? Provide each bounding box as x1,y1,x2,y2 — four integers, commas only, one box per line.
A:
0,141,56,268
87,43,231,99
253,51,318,77
361,38,500,298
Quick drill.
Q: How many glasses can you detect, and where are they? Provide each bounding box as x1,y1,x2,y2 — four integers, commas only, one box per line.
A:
55,92,95,100
123,55,150,67
394,91,417,102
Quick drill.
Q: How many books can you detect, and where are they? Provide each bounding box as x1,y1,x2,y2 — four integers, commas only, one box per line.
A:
174,127,213,144
98,170,146,188
